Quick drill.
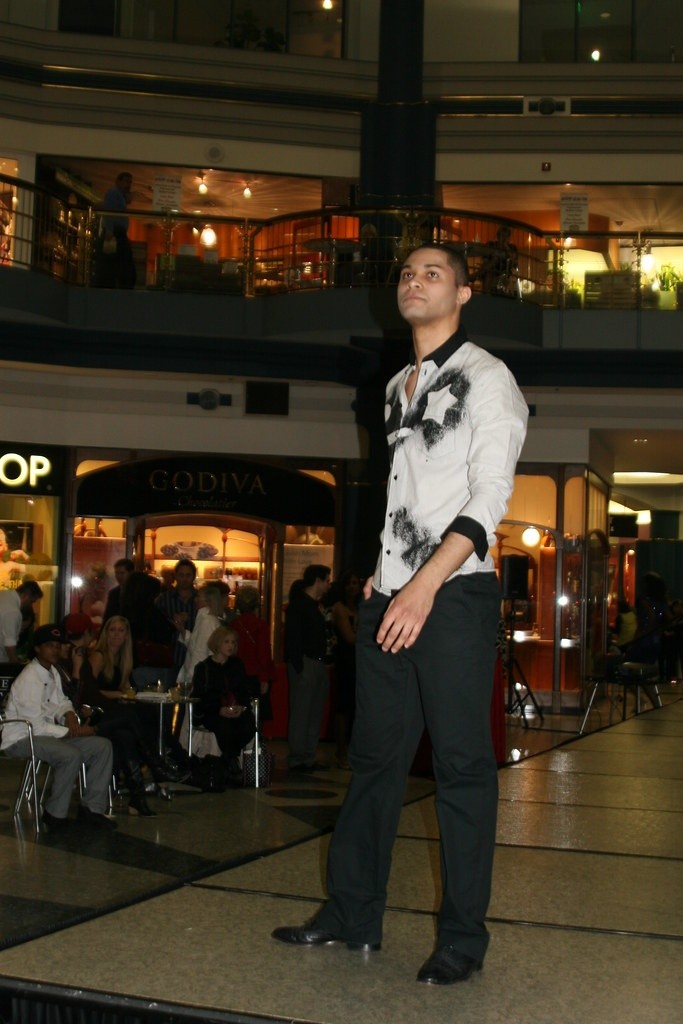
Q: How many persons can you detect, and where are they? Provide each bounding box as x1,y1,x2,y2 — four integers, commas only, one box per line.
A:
174,585,225,683
226,585,271,678
0,581,44,662
0,629,119,833
611,598,683,683
191,627,256,791
88,615,133,691
65,612,97,677
104,172,137,290
102,558,134,617
54,630,191,816
284,563,360,773
156,559,200,686
477,226,519,295
271,242,528,985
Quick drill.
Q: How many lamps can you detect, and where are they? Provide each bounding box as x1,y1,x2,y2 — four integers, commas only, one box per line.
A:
521,525,540,547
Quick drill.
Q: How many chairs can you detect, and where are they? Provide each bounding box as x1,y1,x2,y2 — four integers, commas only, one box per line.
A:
0,662,114,834
185,673,262,789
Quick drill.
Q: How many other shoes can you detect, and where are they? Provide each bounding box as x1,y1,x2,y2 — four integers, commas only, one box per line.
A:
74,805,118,831
42,811,80,837
287,764,321,776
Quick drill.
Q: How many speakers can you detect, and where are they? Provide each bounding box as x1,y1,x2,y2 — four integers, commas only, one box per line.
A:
499,554,528,600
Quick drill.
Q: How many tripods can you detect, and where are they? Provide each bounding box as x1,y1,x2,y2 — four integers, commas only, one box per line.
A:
503,602,544,731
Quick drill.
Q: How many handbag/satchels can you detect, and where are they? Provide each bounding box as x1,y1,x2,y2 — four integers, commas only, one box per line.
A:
243,739,271,787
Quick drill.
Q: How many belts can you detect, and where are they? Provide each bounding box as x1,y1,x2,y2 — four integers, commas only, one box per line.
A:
303,652,328,664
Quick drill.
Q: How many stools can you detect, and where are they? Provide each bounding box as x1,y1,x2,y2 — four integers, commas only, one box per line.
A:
578,659,663,735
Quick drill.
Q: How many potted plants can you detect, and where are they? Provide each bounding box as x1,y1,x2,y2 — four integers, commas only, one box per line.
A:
546,260,683,310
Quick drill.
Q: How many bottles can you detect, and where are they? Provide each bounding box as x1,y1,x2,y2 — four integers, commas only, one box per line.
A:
88,707,104,727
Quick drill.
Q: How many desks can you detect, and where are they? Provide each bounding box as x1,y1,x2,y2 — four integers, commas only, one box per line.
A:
119,679,202,801
302,238,366,289
442,240,488,287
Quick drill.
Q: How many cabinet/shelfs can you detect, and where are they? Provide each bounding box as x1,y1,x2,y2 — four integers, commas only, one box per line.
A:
49,176,92,287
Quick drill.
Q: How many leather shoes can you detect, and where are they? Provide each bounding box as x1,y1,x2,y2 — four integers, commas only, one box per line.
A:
270,923,381,953
418,944,484,987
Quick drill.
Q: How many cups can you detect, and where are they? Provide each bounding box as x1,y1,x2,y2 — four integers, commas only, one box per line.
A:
126,685,136,699
179,681,192,698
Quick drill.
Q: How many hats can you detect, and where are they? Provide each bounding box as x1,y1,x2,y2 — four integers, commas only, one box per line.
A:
32,625,62,643
61,626,77,647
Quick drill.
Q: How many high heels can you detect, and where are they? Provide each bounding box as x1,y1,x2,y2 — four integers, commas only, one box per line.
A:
153,768,197,792
332,753,351,770
127,798,157,818
